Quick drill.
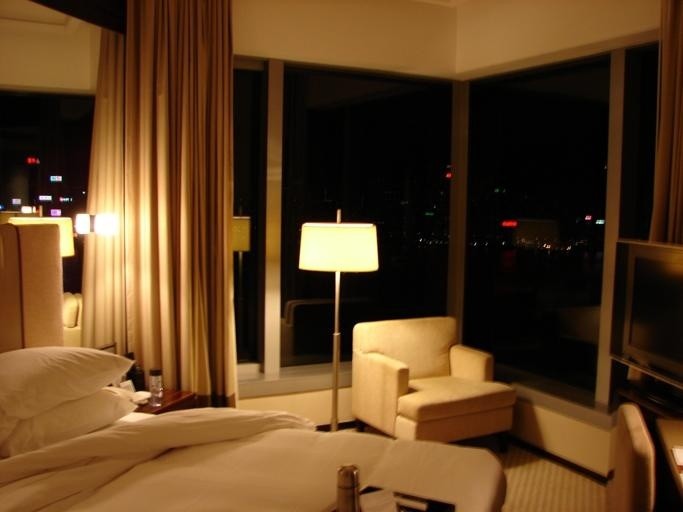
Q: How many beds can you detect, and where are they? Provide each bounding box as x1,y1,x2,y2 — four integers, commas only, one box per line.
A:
0,225,507,511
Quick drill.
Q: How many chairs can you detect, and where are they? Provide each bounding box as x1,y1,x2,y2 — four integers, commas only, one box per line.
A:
607,402,660,512
351,314,518,442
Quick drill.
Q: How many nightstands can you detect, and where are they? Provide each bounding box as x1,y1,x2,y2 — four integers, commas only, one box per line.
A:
133,387,203,414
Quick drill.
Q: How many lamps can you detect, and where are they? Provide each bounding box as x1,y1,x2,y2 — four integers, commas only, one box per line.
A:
298,209,381,434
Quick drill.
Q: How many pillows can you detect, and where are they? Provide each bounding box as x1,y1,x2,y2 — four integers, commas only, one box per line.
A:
0,346,137,458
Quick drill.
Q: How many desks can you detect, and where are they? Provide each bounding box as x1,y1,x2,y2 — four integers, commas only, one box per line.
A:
655,415,683,491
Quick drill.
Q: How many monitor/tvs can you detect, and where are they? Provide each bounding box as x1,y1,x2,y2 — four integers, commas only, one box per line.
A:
609,240,683,409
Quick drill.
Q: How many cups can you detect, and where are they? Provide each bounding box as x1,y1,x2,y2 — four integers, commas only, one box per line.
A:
336,465,360,512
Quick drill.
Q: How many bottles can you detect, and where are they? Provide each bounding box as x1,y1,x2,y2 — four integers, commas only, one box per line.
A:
149,369,163,407
